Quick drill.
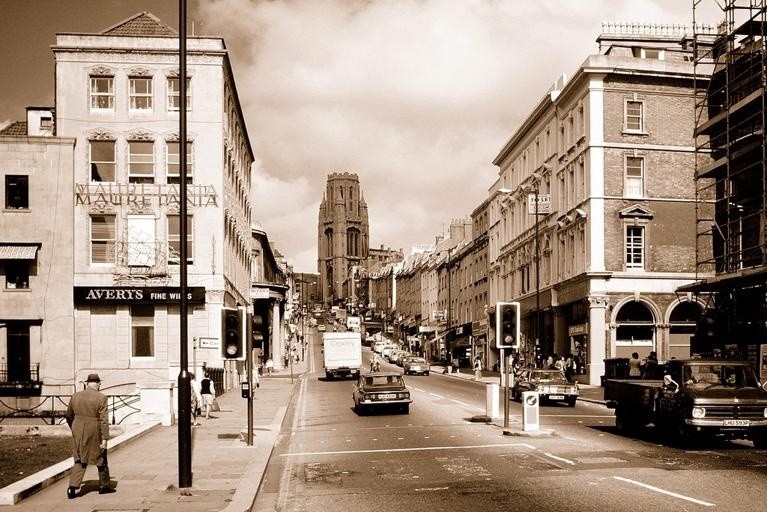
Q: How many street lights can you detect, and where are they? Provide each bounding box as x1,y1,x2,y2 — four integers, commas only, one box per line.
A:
424,247,454,373
328,276,363,315
497,186,543,369
371,274,390,340
301,272,322,362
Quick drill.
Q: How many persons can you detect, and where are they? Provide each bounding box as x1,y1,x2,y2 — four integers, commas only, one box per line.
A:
630,351,659,379
474,358,482,382
63,371,117,499
513,357,518,373
543,353,584,380
199,372,215,419
244,301,422,376
189,372,200,426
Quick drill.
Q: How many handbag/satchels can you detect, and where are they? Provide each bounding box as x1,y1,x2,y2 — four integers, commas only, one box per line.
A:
211,401,219,411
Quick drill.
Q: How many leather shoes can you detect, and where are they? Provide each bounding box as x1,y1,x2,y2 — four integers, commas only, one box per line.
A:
67,487,74,498
99,487,115,494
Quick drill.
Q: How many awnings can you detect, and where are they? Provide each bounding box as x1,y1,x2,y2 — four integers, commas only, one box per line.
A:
429,329,453,342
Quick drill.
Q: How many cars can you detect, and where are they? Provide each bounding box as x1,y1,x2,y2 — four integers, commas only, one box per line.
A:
351,369,414,419
307,303,431,379
510,364,581,409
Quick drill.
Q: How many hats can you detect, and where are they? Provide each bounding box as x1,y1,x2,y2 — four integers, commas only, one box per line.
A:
85,374,103,382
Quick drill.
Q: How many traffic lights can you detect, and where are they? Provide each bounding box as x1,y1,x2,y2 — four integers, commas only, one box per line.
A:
496,303,521,349
219,305,248,362
534,345,544,365
250,313,266,351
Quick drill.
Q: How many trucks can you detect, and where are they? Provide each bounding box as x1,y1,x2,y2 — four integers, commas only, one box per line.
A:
602,356,767,451
